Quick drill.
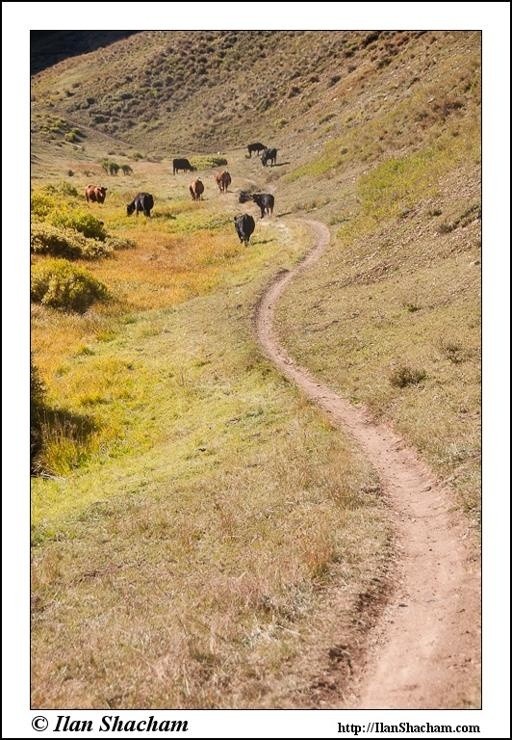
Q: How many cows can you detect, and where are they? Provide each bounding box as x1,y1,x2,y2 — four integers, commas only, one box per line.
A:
188,180,205,200
215,170,232,193
172,158,194,176
247,142,268,157
126,192,154,217
259,147,279,166
234,213,255,246
249,193,274,219
84,184,108,204
